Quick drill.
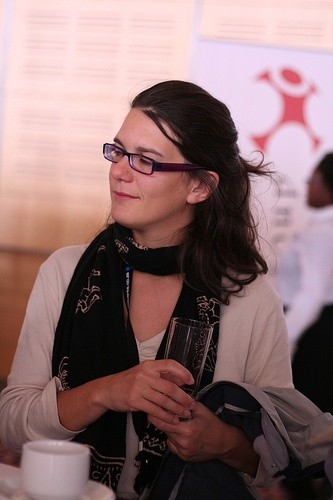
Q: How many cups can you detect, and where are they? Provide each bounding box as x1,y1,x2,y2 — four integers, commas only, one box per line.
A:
19,438,91,500
163,317,213,420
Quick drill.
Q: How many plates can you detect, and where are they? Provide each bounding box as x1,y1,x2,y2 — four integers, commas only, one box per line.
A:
0,464,116,500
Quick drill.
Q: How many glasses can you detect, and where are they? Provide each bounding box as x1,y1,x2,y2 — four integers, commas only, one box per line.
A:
102,143,205,176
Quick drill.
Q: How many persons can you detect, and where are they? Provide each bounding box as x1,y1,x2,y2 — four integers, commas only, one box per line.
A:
0,81,295,500
